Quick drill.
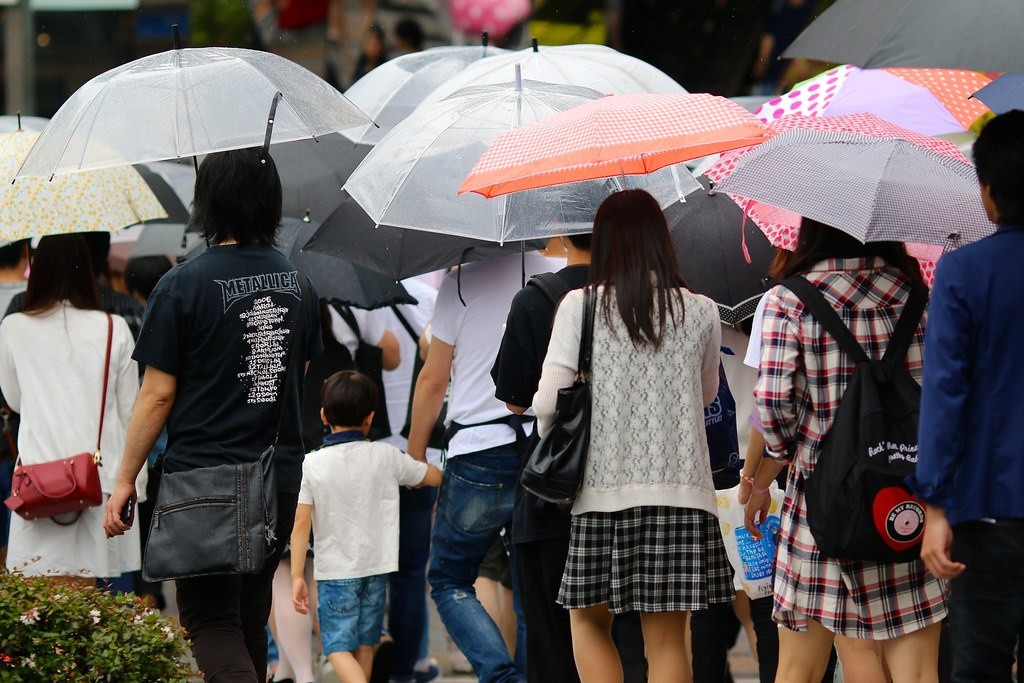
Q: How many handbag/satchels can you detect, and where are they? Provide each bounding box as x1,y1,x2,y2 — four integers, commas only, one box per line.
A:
521,285,597,505
3,451,103,525
143,446,278,583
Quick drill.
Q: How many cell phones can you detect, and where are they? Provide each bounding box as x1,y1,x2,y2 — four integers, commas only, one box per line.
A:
110,498,133,537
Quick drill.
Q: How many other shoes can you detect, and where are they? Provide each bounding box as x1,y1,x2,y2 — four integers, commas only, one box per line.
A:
447,652,474,673
414,665,439,682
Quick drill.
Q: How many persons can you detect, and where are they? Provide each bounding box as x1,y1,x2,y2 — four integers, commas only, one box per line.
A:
395,19,422,55
351,21,387,85
915,109,1024,683
0,148,952,683
758,0,812,96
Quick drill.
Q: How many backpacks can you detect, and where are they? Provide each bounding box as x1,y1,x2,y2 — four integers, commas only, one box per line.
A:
778,274,929,566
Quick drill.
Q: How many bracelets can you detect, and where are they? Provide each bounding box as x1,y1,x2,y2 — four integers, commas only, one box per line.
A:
739,469,755,483
752,482,769,493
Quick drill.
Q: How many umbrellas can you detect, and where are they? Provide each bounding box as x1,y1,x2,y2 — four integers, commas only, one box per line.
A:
0,0,1024,331
